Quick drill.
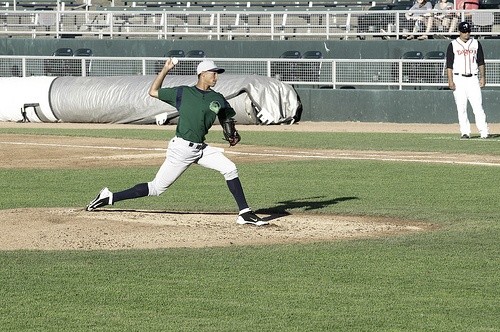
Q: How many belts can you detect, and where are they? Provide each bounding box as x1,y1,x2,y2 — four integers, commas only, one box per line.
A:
172,140,208,149
454,73,478,77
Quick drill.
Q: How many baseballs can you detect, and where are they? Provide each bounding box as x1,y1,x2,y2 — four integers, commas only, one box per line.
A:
172,57,179,65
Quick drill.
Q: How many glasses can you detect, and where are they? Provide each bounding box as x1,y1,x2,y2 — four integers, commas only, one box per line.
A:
462,30,471,33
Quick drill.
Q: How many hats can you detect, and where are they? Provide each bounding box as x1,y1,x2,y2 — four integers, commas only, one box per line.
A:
458,21,471,31
197,60,225,75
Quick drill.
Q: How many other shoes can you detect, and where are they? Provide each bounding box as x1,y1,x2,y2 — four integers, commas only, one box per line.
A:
461,134,470,139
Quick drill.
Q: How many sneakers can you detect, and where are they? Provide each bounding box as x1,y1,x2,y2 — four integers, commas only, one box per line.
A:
86,186,113,211
237,207,269,226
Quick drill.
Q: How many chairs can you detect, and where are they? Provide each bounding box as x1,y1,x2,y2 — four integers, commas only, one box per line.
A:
274,50,322,89
154,50,207,79
44,48,94,77
357,0,500,40
393,52,445,91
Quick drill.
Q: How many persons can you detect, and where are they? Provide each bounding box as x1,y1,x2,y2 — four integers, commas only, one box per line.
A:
446,21,489,139
85,57,270,228
405,0,458,40
